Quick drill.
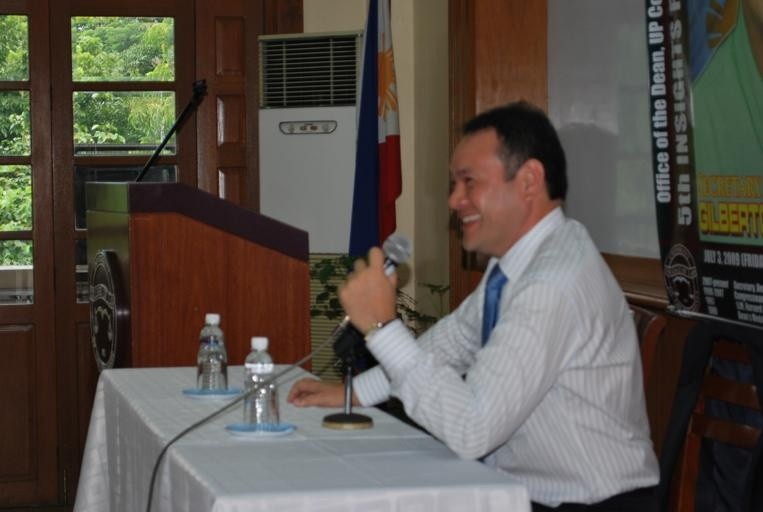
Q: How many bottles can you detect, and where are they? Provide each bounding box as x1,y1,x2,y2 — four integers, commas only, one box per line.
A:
195,312,229,393
242,336,280,430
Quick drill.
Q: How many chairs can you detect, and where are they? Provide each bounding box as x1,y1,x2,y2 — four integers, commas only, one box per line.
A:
628,303,666,388
657,322,763,512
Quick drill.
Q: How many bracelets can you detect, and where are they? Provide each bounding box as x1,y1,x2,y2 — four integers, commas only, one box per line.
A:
363,317,398,343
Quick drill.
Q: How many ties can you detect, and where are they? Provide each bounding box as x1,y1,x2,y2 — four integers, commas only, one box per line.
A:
483,263,507,347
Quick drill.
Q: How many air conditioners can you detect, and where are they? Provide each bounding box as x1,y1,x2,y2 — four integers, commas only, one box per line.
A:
258,30,363,380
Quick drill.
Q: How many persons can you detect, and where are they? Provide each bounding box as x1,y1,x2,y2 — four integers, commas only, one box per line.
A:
288,101,662,512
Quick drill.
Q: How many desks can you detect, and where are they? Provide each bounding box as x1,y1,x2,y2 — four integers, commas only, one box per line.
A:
74,363,531,512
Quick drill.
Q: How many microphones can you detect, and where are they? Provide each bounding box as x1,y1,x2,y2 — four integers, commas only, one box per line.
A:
332,232,412,338
